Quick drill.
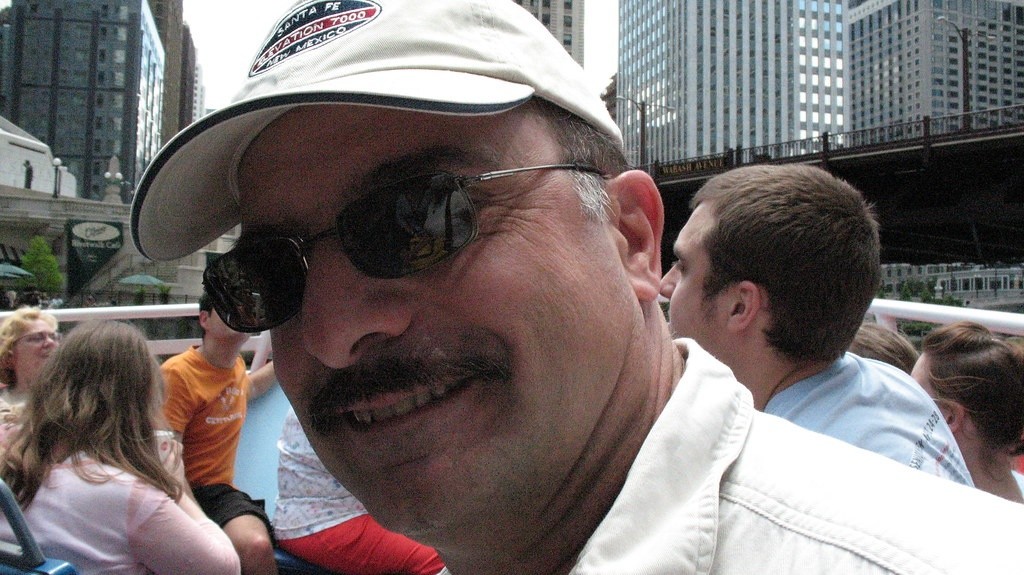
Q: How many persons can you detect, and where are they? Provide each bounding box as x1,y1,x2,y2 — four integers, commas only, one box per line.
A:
0,162,1024,575
129,1,1024,575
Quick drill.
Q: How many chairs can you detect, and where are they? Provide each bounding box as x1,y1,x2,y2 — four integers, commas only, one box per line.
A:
233,379,333,575
0,479,79,575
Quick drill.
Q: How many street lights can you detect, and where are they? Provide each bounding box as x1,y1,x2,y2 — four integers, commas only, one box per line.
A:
616,95,677,165
937,14,997,133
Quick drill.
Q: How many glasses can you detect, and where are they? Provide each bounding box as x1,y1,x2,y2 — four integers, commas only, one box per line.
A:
15,331,63,344
202,163,611,334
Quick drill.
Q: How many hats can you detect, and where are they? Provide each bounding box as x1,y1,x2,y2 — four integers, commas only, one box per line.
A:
129,0,625,265
